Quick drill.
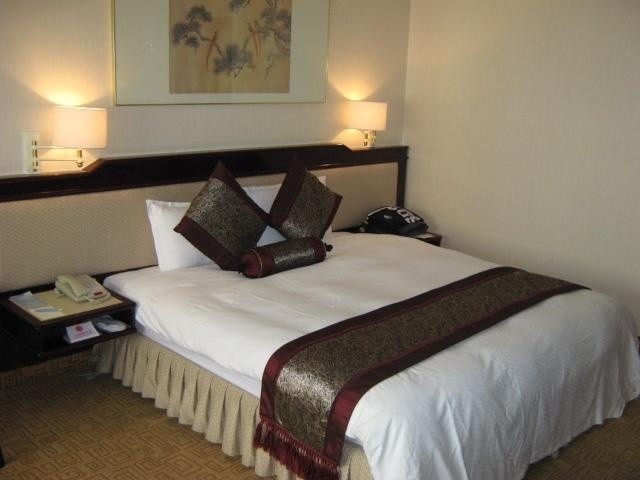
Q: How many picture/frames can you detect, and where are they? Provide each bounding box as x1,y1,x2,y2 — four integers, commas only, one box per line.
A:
107,0,330,106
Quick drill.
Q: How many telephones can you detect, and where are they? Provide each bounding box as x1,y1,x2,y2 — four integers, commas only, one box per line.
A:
55,272,111,304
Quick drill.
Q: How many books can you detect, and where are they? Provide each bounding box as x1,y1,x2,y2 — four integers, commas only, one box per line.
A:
64,318,101,345
8,290,46,311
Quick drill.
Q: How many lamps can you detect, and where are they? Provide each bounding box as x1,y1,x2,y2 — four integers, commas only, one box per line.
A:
352,101,388,147
30,106,109,173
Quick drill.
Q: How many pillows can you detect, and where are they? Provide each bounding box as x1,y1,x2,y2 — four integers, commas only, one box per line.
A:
145,159,345,278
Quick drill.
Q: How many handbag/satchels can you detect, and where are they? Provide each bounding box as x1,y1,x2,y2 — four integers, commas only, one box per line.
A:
361,204,429,238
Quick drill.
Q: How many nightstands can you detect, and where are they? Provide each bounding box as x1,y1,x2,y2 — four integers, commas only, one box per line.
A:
346,224,442,248
0,276,138,367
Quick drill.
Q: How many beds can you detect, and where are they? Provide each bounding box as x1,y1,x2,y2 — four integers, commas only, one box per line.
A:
0,142,632,480
